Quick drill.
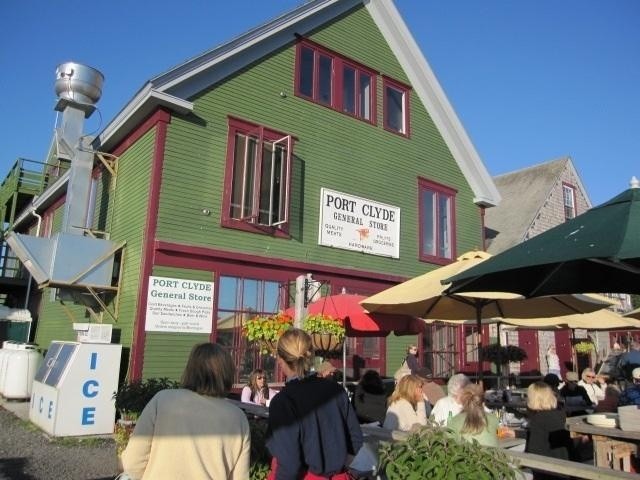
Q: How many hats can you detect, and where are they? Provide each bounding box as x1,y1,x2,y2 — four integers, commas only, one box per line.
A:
544,373,563,387
566,371,579,382
632,367,640,379
446,374,469,396
416,366,433,379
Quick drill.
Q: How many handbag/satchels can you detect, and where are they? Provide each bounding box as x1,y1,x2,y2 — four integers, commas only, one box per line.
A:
393,358,412,384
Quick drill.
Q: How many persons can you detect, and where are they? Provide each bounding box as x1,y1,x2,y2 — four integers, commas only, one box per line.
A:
417,367,445,407
543,374,563,392
524,382,571,460
122,344,251,480
241,369,276,418
577,368,607,404
617,367,640,406
352,369,387,428
316,362,337,381
449,383,499,449
383,375,427,433
264,328,362,480
545,343,562,380
558,372,592,407
429,374,490,431
403,343,419,376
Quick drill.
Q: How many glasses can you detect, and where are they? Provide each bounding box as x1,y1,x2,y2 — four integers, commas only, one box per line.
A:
257,376,266,380
587,376,596,379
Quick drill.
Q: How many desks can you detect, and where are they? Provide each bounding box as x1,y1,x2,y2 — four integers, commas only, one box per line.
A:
565,412,640,472
485,395,528,419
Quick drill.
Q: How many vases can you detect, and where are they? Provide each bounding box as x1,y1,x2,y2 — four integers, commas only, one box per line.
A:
309,333,342,354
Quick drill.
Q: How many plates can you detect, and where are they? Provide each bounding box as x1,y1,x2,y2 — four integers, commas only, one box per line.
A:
587,413,616,428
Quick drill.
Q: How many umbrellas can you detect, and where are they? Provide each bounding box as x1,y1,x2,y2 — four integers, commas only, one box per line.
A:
360,248,620,382
441,176,640,300
271,293,425,392
415,310,640,349
623,307,640,321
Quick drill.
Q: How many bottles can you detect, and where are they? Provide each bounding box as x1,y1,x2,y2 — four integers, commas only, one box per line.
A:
447,411,453,427
504,384,512,402
518,391,527,411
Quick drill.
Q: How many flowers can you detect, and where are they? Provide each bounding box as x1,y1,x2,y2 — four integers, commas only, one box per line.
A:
303,312,347,344
240,313,294,342
